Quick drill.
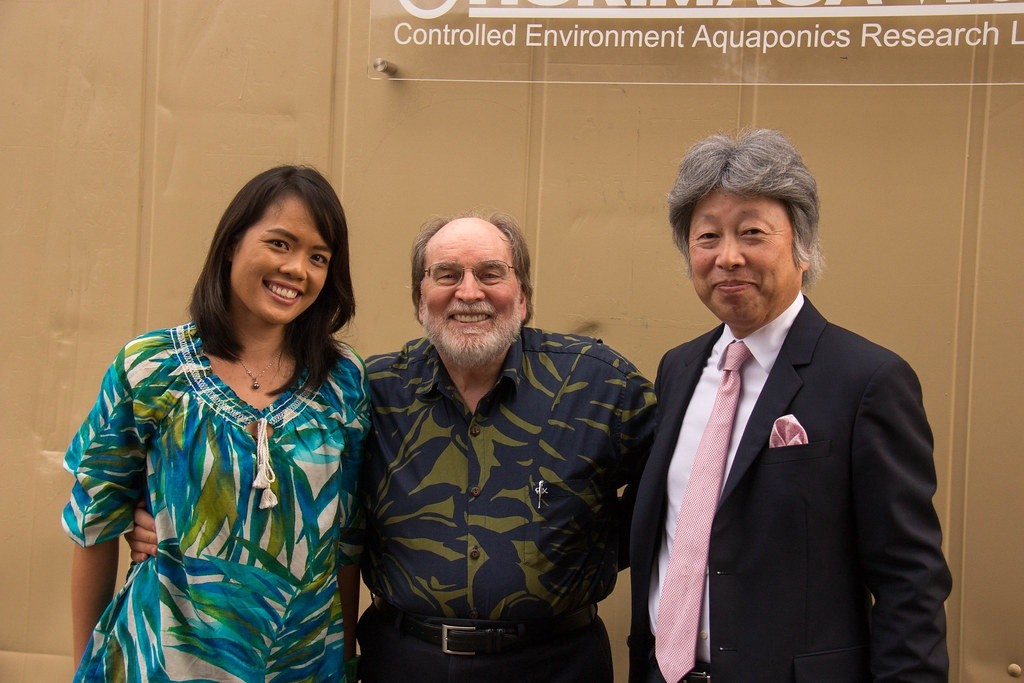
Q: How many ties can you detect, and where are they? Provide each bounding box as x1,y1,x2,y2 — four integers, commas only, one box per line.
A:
654,342,752,683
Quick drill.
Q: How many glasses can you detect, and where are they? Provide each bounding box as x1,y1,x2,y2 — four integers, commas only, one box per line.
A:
424,259,516,287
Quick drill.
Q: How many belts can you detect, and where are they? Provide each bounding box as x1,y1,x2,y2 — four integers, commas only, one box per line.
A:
374,596,597,655
679,670,710,683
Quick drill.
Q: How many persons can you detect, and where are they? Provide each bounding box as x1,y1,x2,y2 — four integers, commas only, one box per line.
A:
626,128,953,683
126,215,656,683
62,167,372,683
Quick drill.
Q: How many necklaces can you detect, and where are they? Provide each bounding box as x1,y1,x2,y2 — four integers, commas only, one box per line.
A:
238,352,281,389
